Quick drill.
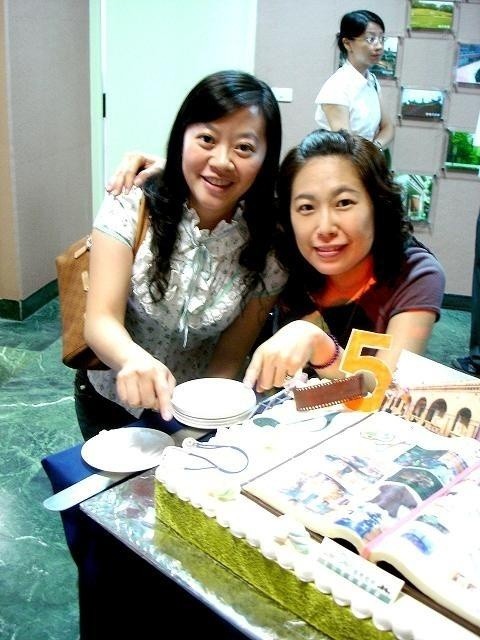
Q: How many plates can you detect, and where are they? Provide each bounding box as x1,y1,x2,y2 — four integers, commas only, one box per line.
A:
81,426,174,473
171,378,257,430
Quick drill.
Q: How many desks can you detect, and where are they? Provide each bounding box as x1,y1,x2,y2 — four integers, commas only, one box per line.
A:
44,369,480,631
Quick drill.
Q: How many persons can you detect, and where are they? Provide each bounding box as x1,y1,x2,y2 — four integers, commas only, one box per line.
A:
315,10,396,163
75,69,299,445
104,128,446,395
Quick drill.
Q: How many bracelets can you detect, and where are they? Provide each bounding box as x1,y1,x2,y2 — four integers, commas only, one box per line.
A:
308,333,339,370
374,139,383,149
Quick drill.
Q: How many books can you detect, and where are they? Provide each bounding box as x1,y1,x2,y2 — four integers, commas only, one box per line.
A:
240,410,479,629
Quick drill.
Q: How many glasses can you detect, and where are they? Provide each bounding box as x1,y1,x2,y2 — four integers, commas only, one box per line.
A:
352,34,386,43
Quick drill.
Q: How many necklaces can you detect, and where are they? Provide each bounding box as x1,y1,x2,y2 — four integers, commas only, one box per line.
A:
308,276,372,349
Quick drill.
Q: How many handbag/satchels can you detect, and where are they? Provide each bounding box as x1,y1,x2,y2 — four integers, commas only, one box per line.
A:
54,191,148,371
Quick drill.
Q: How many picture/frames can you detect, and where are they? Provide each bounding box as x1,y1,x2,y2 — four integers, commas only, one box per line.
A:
449,40,477,93
388,171,439,232
442,131,477,180
407,3,462,34
396,83,445,123
337,36,403,83
